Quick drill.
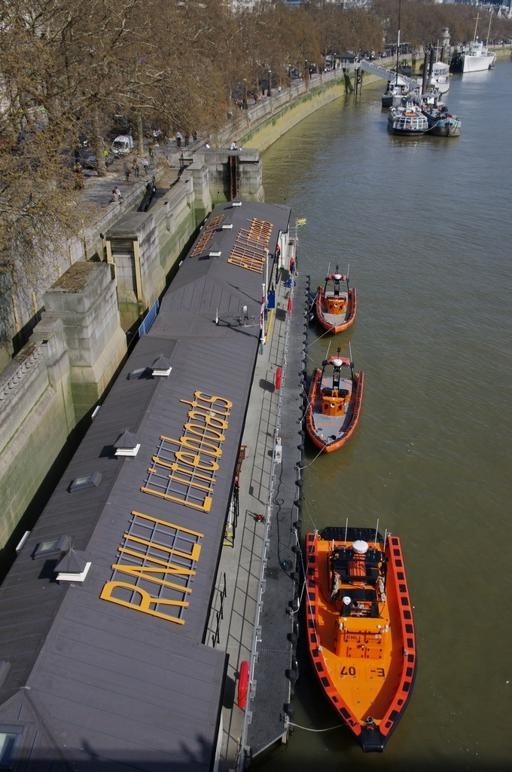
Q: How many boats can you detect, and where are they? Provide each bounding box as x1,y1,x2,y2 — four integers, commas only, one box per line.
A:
315,273,357,334
382,0,497,137
305,526,418,754
306,357,364,456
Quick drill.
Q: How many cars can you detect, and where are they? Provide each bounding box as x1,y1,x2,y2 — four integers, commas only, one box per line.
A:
78,135,134,169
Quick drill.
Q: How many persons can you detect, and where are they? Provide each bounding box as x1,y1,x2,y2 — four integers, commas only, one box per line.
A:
142,156,148,174
132,158,139,177
230,141,238,150
176,132,182,147
109,186,120,202
